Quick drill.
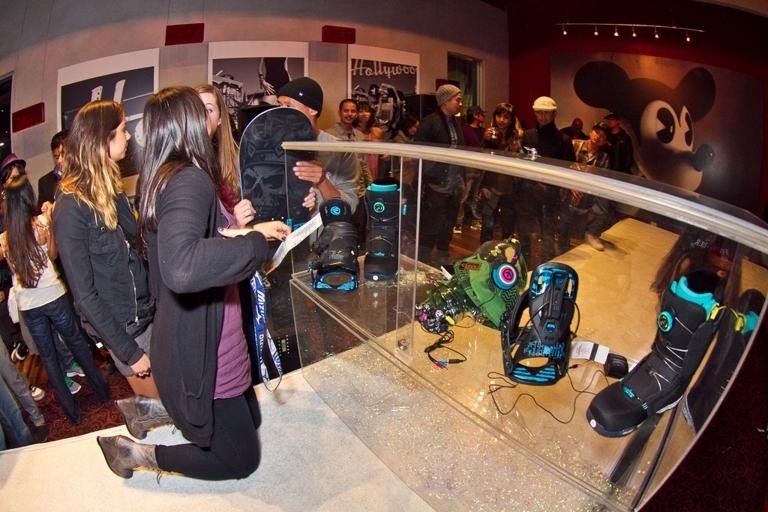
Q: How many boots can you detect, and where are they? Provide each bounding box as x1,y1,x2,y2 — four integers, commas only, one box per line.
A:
586,266,724,437
363,177,398,281
98,435,161,478
115,396,174,439
308,200,359,292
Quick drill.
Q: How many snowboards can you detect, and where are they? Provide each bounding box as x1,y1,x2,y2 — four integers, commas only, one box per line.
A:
240,107,318,384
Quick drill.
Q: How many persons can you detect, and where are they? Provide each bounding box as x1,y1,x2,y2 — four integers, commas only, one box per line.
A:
258,58,292,96
414,84,466,264
275,78,419,258
649,220,736,309
195,85,256,228
96,86,294,479
456,97,632,267
0,128,116,450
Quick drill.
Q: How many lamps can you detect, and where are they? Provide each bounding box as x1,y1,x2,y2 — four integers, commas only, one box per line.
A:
561,23,690,42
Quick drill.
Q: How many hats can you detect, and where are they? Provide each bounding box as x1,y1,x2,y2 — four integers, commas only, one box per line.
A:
0,153,26,170
533,96,557,111
437,85,461,106
276,77,322,111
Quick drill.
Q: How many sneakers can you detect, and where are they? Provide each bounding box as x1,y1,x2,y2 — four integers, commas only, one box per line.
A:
585,232,604,250
470,222,482,231
11,344,84,443
453,224,462,233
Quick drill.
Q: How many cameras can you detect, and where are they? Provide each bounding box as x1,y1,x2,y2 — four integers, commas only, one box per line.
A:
490,129,501,145
